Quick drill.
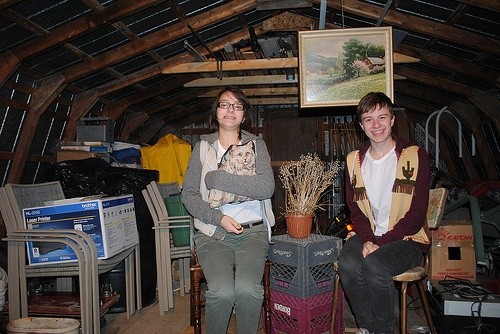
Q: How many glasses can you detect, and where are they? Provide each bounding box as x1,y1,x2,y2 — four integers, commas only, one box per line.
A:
216,100,247,111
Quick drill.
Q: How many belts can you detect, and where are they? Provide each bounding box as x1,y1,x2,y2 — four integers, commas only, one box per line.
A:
236,221,264,229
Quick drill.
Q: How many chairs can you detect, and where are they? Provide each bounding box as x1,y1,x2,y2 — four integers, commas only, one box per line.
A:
329,187,448,334
329,187,449,334
0,181,142,334
142,181,196,313
190,217,272,334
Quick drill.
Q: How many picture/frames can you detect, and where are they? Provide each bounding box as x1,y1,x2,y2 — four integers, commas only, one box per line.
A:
298,26,394,108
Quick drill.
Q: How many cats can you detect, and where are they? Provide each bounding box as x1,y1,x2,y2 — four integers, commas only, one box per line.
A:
208,140,256,208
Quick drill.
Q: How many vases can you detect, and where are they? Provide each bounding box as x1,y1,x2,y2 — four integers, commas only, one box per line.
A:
286,213,314,238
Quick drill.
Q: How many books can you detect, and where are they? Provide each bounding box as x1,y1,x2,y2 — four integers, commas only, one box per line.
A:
58,141,112,153
324,116,364,222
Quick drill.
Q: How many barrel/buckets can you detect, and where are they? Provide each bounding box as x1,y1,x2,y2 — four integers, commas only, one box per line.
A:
164,192,190,247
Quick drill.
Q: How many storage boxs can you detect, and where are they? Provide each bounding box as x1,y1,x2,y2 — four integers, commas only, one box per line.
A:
56,151,111,166
268,234,345,334
424,220,476,284
21,194,140,267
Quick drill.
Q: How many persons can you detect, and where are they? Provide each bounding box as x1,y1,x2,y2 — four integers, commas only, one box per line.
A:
338,92,432,334
182,88,276,334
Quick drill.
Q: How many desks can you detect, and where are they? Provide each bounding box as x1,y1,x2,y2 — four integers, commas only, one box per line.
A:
426,276,500,334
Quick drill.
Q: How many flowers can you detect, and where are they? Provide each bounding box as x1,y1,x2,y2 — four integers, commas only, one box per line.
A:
276,152,342,214
